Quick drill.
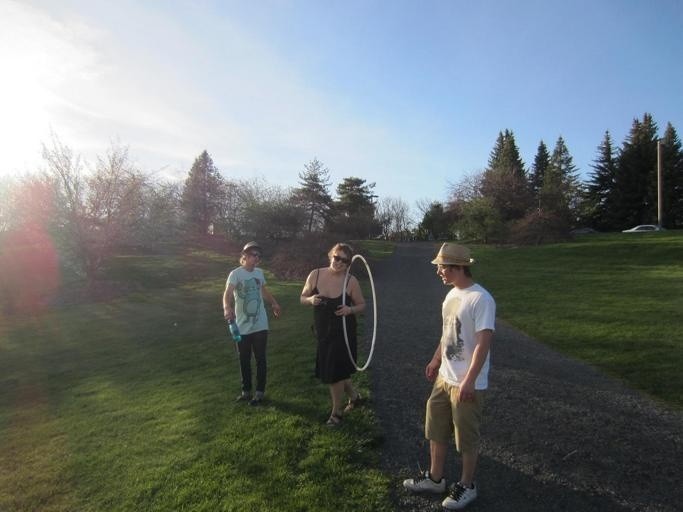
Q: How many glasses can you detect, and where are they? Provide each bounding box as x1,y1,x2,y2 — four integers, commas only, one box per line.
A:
334,256,347,263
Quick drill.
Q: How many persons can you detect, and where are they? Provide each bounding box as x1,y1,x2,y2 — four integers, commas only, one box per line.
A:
300,242,366,426
222,242,282,405
403,243,497,509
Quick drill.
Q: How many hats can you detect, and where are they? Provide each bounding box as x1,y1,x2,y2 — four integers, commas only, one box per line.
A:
431,243,475,266
241,242,263,255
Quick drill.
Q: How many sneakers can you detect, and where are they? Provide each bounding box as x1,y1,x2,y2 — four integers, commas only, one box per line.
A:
442,482,477,509
235,395,264,406
403,470,446,493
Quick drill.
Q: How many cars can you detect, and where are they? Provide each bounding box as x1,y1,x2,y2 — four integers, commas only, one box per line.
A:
621,224,667,233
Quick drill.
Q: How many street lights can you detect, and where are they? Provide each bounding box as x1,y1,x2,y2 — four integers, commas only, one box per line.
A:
652,134,667,226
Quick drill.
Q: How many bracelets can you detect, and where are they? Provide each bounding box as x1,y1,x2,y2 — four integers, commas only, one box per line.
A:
349,306,355,314
224,304,231,308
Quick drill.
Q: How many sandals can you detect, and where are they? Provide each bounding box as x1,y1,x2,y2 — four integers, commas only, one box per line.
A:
325,413,341,425
344,394,361,413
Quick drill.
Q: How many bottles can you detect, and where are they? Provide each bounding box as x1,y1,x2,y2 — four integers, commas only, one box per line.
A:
226,319,242,343
